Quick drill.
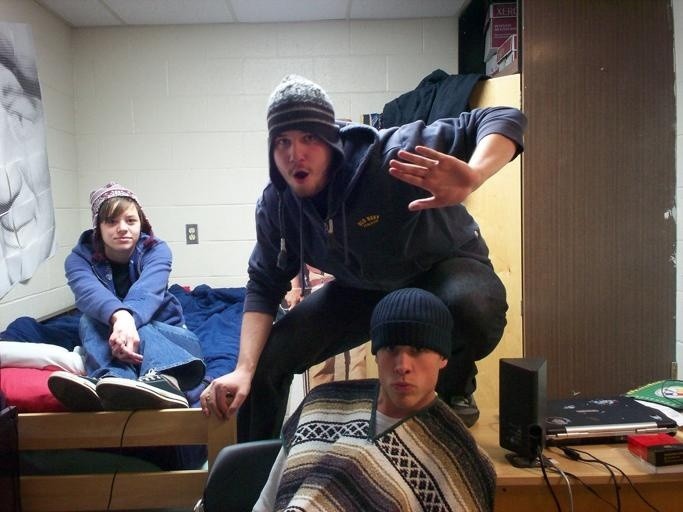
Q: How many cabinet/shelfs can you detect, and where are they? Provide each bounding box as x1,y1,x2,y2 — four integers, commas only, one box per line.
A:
465,1,674,414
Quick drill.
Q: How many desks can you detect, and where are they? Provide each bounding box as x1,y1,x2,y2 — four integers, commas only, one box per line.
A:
462,416,682,512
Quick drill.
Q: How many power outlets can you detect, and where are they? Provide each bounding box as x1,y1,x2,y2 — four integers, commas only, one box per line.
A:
185,224,199,245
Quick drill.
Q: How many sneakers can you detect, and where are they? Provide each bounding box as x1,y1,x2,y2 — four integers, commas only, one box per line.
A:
97,374,190,409
47,368,100,412
440,388,479,429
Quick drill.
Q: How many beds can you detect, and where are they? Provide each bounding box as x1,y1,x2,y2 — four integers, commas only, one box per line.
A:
2,279,292,511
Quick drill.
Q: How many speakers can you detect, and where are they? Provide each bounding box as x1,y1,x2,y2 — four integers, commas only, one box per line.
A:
500,356,547,465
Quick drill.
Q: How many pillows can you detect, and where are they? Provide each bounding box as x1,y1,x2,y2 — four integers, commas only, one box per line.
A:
1,367,84,413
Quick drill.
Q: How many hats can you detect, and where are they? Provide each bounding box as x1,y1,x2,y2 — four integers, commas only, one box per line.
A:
368,286,453,360
88,181,155,263
264,73,346,195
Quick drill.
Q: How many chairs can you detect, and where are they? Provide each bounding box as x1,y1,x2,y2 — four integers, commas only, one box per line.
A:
188,438,292,512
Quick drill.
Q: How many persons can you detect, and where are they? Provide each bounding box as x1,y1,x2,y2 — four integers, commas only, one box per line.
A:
201,72,525,446
43,184,207,410
0,27,56,288
252,287,498,512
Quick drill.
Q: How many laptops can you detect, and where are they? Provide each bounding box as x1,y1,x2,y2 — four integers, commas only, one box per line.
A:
548,395,677,444
